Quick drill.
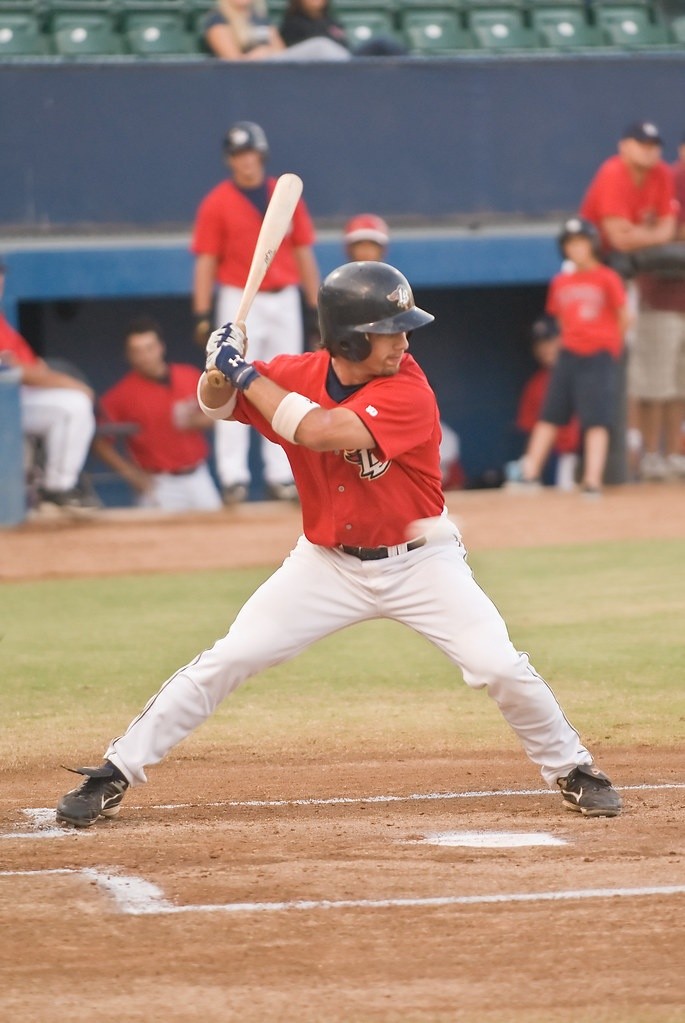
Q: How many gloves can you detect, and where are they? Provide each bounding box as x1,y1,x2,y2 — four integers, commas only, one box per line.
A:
205,345,260,392
205,322,245,357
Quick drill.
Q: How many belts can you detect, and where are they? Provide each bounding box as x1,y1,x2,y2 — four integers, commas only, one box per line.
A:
337,537,426,561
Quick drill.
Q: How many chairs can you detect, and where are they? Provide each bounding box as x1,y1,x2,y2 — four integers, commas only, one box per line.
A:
39,356,161,510
0,0,685,53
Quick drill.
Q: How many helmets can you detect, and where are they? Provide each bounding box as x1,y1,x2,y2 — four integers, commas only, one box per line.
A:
317,260,435,362
345,213,387,245
223,122,268,154
622,122,662,146
557,220,601,260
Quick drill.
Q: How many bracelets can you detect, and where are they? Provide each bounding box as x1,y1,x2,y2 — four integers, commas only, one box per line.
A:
195,310,210,325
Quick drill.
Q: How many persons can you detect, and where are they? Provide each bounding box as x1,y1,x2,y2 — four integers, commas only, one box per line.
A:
631,131,685,481
194,120,323,504
501,215,635,499
278,0,407,56
204,0,355,61
91,318,226,511
343,213,389,261
0,261,106,513
517,319,583,454
56,261,622,830
576,122,676,477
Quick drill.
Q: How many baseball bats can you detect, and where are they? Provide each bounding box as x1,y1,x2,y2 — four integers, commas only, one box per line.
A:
207,173,304,389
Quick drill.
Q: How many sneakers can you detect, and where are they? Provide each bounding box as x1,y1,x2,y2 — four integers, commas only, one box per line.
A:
556,765,622,816
56,766,126,826
